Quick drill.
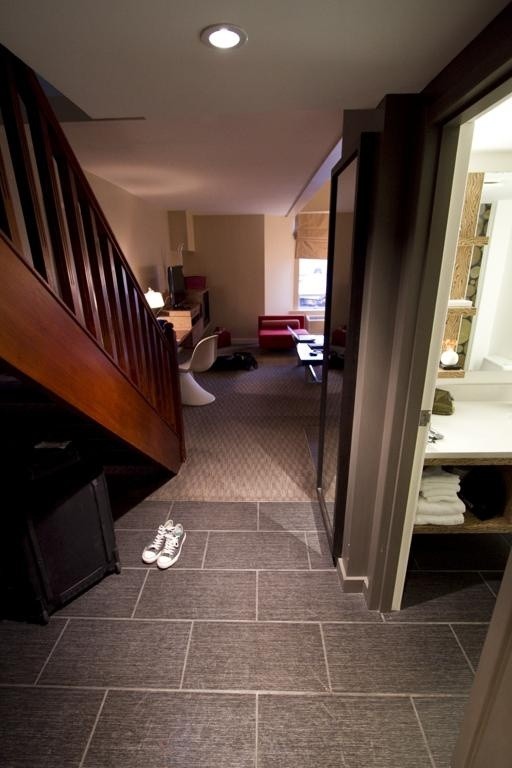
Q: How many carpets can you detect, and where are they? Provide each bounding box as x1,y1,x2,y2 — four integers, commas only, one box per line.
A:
130,337,323,502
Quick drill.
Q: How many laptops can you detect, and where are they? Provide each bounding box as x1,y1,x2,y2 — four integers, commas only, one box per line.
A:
287,325,316,342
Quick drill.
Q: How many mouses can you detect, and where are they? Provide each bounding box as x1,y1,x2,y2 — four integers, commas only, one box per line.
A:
309,352,317,356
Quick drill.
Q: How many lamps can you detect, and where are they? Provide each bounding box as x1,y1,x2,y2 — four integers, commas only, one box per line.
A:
144,287,164,309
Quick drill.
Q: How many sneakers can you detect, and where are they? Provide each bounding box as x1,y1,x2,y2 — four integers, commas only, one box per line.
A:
156,523,187,570
143,518,173,564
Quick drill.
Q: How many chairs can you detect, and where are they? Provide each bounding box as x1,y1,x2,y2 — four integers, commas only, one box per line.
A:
258,315,309,355
176,334,218,407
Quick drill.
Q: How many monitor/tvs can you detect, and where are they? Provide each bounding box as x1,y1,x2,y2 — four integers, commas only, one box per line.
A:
165,265,187,308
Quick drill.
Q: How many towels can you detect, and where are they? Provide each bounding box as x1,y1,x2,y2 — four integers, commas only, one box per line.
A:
432,388,453,416
415,465,466,526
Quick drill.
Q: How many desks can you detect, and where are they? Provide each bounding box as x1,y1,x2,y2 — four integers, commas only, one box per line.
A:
157,303,203,346
175,329,191,345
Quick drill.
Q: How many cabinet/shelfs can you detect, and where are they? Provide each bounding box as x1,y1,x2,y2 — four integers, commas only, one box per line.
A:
438,239,489,378
413,400,511,533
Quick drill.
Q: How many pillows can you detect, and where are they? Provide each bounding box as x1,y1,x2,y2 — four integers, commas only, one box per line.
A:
262,319,300,329
